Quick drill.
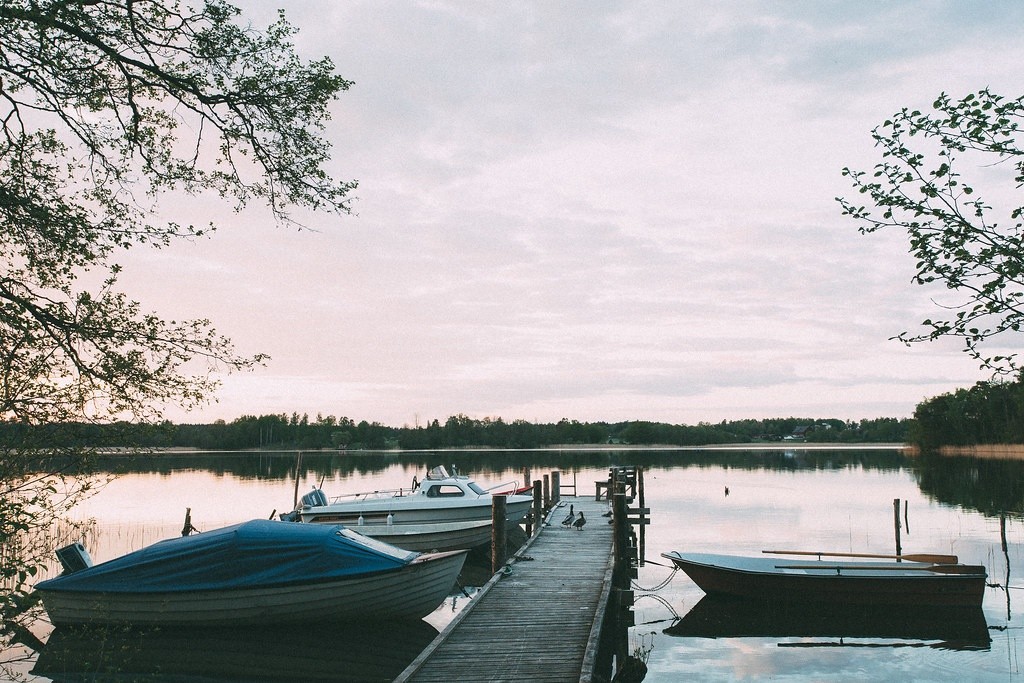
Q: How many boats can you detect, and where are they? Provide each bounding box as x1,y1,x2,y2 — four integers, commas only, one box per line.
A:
661,594,995,654
660,548,987,607
337,517,510,552
277,464,536,539
26,616,441,683
32,514,470,628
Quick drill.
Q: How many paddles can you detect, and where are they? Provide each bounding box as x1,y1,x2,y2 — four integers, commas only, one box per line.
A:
773,565,986,576
777,638,993,651
762,549,959,565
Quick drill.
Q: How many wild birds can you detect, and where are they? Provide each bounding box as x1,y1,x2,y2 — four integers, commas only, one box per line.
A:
572,511,586,531
561,504,575,529
